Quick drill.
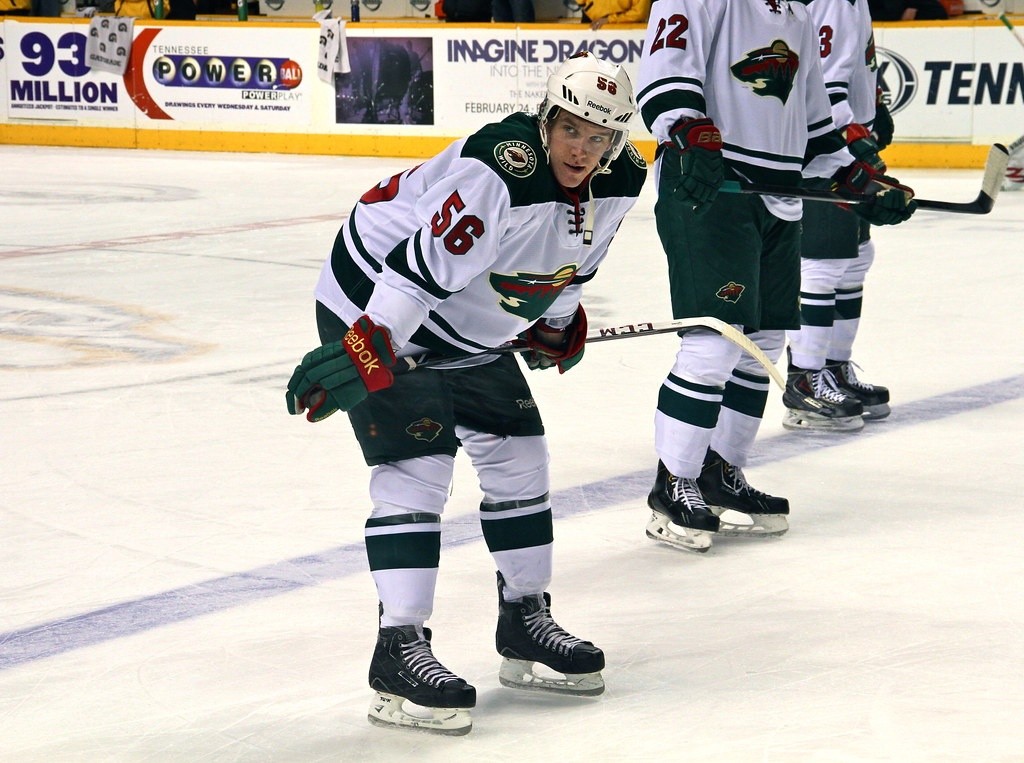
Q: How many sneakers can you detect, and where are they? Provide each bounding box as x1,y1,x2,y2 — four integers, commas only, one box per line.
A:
825,360,890,421
782,345,864,432
646,459,719,552
496,570,606,698
367,601,476,737
697,444,790,538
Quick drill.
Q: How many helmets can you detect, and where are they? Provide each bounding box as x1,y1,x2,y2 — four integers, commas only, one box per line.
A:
540,50,634,160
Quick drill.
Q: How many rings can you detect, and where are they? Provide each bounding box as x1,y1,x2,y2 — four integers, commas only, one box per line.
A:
596,21,599,25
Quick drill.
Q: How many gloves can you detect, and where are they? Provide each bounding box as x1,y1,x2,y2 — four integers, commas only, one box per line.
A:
840,123,886,174
669,115,725,214
515,303,588,375
286,316,396,422
831,159,916,227
872,87,895,151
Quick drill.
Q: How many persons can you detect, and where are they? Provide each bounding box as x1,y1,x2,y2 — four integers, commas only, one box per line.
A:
980,0,1024,192
0,0,963,51
285,57,647,737
783,0,895,434
637,0,917,554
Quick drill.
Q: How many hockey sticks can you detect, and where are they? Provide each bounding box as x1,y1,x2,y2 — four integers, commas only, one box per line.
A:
718,143,1011,215
305,315,785,393
